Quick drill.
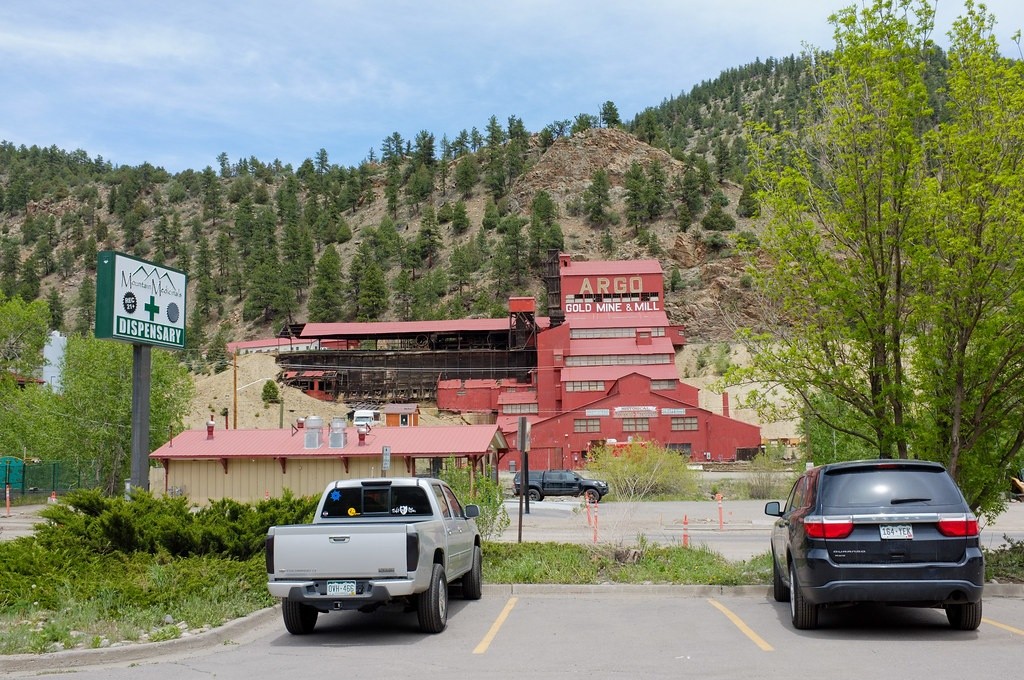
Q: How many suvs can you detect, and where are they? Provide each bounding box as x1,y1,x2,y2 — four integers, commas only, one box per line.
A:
764,459,986,631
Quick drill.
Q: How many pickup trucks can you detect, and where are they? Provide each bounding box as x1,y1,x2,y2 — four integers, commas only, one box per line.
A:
266,476,484,634
512,470,609,504
